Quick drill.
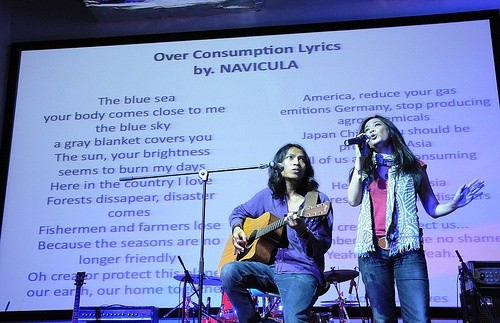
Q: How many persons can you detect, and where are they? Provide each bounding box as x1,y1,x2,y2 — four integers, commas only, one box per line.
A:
220,143,333,323
347,115,485,323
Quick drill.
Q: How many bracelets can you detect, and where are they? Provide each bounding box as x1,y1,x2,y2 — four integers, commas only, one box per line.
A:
300,227,310,237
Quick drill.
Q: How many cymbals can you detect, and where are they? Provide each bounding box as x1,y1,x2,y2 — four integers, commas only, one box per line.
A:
323,269,359,284
173,273,222,287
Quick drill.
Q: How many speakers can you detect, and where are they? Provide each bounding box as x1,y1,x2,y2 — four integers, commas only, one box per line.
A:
460,261,500,323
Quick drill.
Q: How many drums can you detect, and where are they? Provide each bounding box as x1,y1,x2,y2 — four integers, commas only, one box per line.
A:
309,312,334,323
216,311,240,323
202,314,218,323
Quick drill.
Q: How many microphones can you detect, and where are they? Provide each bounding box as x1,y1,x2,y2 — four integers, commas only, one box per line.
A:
269,162,284,172
349,267,356,294
344,134,371,146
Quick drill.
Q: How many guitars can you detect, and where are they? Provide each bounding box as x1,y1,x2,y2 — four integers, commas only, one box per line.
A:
217,200,332,279
71,271,88,323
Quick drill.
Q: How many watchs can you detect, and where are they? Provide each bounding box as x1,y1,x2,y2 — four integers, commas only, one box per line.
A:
355,168,362,175
